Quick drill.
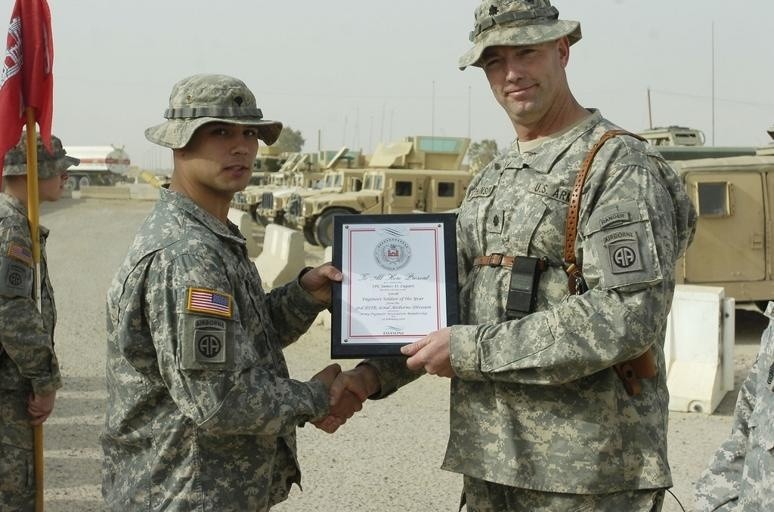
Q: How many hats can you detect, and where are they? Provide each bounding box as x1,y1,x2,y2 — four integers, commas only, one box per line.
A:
1,129,81,180
143,71,284,151
457,0,582,71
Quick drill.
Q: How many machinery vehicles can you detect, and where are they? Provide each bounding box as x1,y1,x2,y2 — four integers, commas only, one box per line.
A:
228,137,477,248
636,127,774,312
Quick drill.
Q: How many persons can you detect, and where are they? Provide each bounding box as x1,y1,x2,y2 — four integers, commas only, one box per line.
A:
98,71,362,512
0,130,79,512
693,303,772,511
310,0,698,510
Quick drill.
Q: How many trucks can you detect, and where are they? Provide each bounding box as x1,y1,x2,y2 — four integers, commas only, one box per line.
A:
63,146,130,191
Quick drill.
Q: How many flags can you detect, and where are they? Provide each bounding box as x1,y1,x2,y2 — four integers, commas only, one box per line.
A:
0,0,57,190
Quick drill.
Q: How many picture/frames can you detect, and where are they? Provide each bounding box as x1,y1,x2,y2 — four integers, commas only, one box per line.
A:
330,213,458,360
